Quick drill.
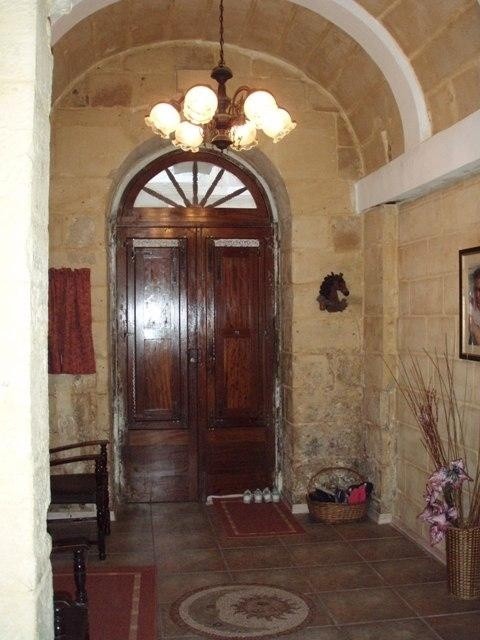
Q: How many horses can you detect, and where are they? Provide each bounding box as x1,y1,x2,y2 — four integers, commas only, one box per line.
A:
320,272,349,303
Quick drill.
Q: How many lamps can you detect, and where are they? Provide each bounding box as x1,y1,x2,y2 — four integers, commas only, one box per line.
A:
145,0,298,153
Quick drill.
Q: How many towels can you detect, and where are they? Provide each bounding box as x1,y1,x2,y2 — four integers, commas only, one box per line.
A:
48,266,97,375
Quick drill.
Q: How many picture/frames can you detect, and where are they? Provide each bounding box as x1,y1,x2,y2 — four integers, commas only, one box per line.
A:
458,246,480,362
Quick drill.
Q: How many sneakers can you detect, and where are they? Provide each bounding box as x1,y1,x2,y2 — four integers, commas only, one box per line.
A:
272,488,280,502
263,487,272,503
243,489,253,504
254,489,263,503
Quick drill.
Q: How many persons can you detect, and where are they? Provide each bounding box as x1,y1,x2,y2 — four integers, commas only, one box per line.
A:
470,267,480,346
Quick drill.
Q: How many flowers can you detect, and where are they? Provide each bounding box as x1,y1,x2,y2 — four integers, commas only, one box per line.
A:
382,334,480,547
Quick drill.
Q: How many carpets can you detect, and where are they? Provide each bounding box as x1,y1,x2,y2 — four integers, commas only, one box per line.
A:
213,499,307,541
53,566,157,640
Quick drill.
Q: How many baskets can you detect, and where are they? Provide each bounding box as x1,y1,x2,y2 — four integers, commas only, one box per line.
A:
444,518,479,600
306,467,371,525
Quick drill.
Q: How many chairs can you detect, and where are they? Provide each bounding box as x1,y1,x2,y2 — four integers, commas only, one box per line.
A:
47,537,91,640
47,439,111,560
